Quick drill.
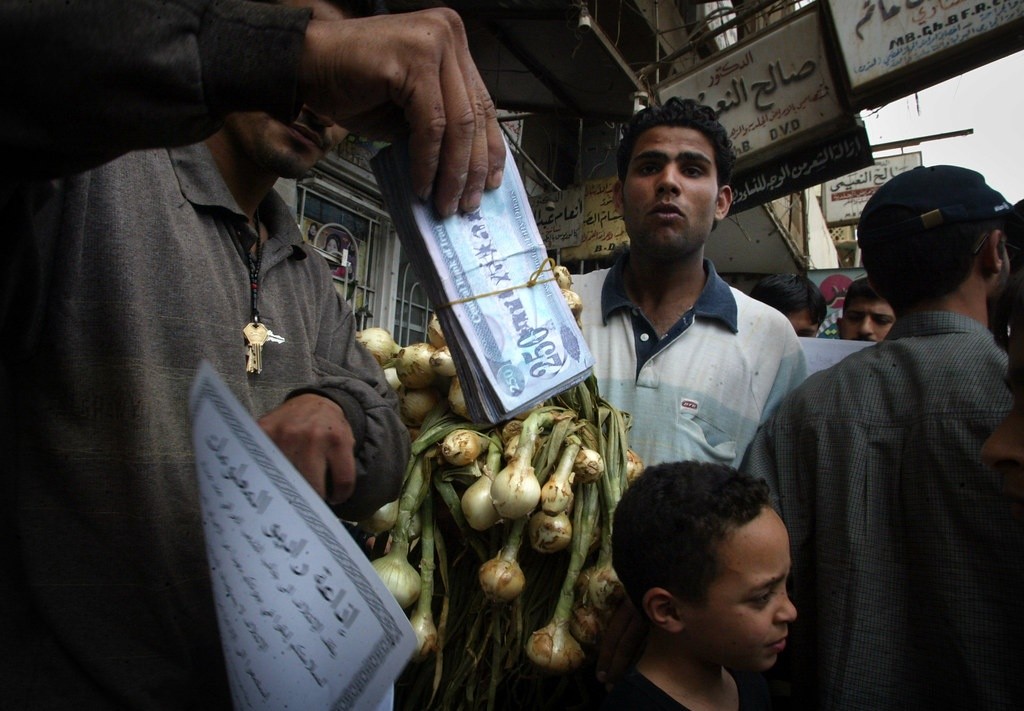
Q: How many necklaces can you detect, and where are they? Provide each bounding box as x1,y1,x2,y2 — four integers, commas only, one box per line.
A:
238,206,260,316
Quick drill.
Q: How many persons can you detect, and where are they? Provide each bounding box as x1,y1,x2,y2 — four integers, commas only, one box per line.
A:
570,97,806,472
980,201,1024,522
306,222,319,241
739,164,1024,711
0,0,507,710
752,274,896,343
602,461,797,711
322,233,352,279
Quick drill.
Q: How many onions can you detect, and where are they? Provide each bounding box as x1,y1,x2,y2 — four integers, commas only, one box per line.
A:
356,263,644,683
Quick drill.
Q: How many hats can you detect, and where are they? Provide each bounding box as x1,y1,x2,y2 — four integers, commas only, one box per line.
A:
857,165,1024,253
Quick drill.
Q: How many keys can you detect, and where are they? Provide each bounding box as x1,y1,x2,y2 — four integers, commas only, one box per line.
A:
240,313,286,375
